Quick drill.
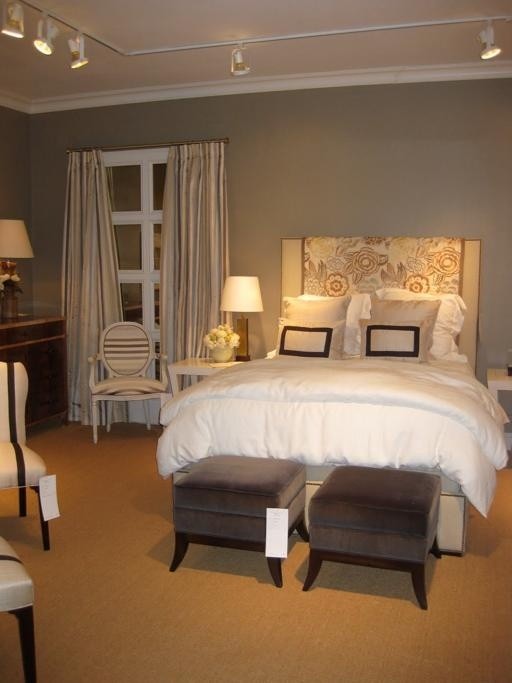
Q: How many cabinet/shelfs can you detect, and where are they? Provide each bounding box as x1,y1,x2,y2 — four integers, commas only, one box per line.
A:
0,313,69,441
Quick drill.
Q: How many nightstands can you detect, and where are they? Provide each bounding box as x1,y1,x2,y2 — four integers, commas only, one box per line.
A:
168,355,248,398
486,367,512,450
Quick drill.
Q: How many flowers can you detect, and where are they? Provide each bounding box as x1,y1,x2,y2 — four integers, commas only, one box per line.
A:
202,324,242,348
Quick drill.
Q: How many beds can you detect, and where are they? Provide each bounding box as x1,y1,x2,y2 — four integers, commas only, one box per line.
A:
156,234,482,558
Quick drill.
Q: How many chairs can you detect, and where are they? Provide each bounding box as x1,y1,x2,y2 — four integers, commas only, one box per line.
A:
0,359,50,550
0,537,36,683
87,322,171,445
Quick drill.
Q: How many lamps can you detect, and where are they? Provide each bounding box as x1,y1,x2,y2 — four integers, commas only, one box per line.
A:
219,275,264,360
0,218,35,318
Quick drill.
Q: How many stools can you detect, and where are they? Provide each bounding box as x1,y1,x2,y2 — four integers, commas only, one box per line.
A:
168,453,309,588
302,464,442,609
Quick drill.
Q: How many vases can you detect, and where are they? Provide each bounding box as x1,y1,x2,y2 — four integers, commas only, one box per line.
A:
209,347,233,362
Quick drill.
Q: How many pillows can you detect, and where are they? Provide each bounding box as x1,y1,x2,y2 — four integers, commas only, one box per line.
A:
376,286,468,363
283,294,352,320
357,317,430,364
275,317,346,362
297,293,371,356
370,294,441,351
